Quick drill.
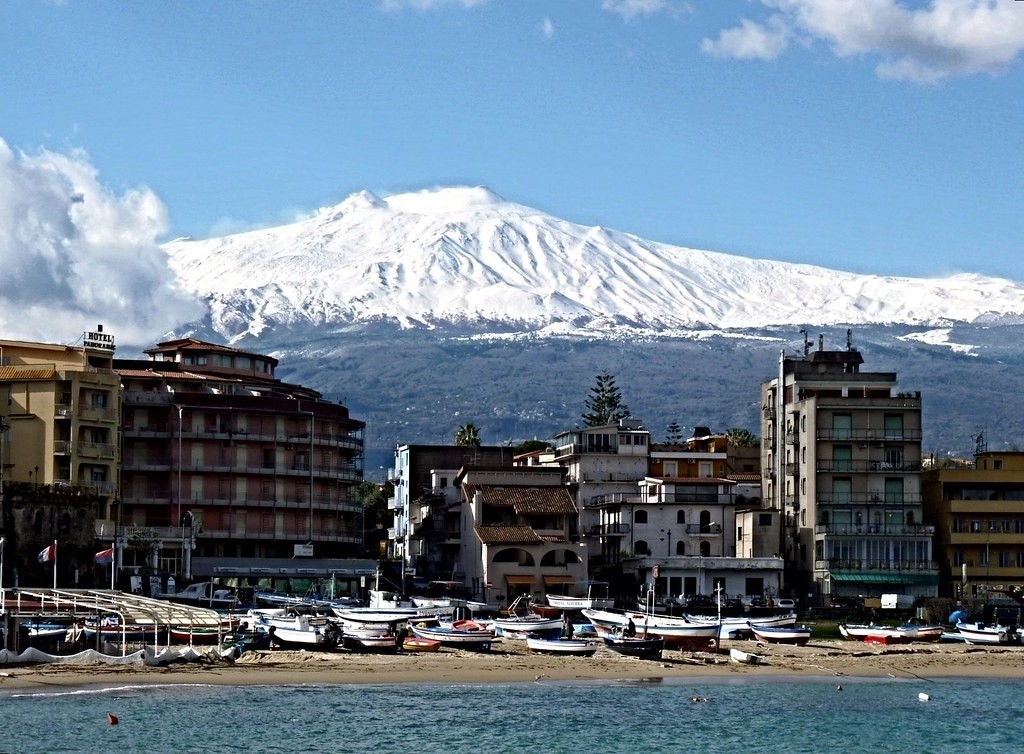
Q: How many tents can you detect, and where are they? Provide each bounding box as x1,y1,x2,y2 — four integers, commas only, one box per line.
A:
191,558,382,610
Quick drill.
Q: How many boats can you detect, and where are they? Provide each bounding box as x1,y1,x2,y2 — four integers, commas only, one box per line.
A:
684,612,801,637
523,634,600,657
839,611,1024,644
747,619,813,645
602,632,664,660
15,563,603,655
581,604,722,648
545,592,617,610
729,648,763,664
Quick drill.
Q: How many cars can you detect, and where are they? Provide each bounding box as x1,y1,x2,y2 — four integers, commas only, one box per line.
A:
749,598,771,609
777,599,797,611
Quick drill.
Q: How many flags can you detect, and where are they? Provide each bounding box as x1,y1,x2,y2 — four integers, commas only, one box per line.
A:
95,548,112,564
38,543,56,564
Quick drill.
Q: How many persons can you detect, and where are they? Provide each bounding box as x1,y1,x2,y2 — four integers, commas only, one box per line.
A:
954,618,962,633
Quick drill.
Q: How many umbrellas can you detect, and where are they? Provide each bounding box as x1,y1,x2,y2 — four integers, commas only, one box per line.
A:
948,611,968,624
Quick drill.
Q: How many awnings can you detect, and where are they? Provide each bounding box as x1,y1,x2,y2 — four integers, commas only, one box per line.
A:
544,576,573,584
505,575,537,584
